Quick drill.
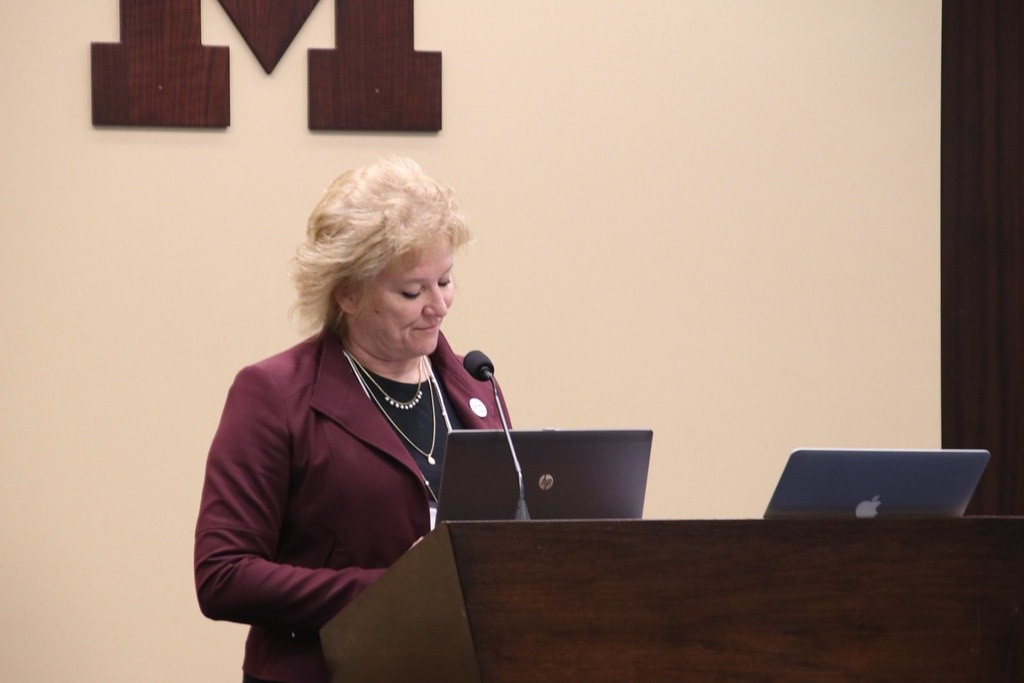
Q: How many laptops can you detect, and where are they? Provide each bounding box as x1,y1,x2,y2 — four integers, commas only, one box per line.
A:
763,449,991,517
436,429,653,522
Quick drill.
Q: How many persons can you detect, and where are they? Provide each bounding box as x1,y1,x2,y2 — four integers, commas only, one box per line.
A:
194,155,514,683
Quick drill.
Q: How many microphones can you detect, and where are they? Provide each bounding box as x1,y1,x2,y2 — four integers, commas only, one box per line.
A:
463,350,531,520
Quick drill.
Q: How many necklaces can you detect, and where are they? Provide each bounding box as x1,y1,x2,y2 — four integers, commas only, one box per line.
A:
341,345,453,465
